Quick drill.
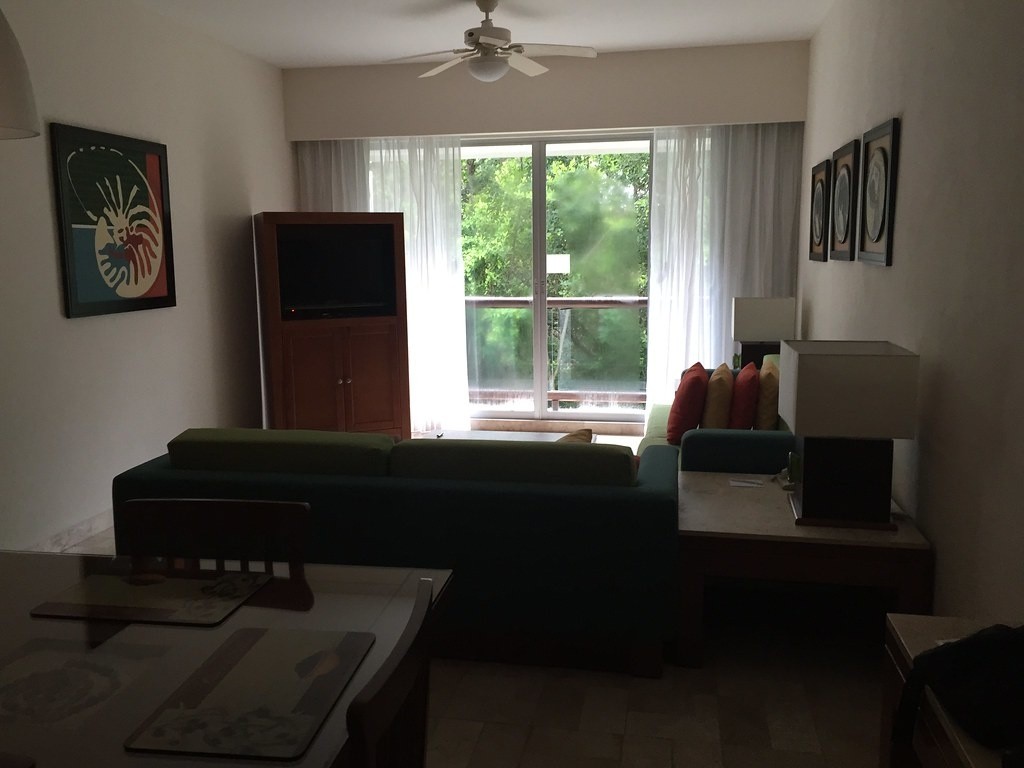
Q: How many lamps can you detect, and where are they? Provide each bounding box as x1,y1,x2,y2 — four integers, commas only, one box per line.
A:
466,48,509,83
0,10,41,141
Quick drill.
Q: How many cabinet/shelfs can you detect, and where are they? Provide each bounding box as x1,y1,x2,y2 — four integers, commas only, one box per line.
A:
253,212,412,441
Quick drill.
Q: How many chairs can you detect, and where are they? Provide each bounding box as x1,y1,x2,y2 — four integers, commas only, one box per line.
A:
121,498,312,565
345,576,433,768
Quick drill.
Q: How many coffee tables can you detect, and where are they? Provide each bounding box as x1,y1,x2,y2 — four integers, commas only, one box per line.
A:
414,431,597,443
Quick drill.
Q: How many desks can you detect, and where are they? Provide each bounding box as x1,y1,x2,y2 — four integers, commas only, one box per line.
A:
0,548,457,768
879,611,1024,768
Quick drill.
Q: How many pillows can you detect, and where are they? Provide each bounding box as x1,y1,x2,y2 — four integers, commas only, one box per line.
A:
553,428,593,443
666,361,779,444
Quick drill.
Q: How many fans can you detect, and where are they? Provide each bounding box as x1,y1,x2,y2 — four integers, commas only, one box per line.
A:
418,0,599,78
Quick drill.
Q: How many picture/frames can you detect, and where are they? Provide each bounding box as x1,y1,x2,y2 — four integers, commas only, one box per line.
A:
857,117,900,267
829,137,860,262
49,122,177,318
808,159,829,263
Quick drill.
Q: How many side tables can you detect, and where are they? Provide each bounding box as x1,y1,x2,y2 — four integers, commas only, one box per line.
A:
675,470,934,669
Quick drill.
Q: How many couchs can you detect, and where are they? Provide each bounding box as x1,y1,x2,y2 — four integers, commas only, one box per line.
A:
112,426,680,681
638,354,794,475
